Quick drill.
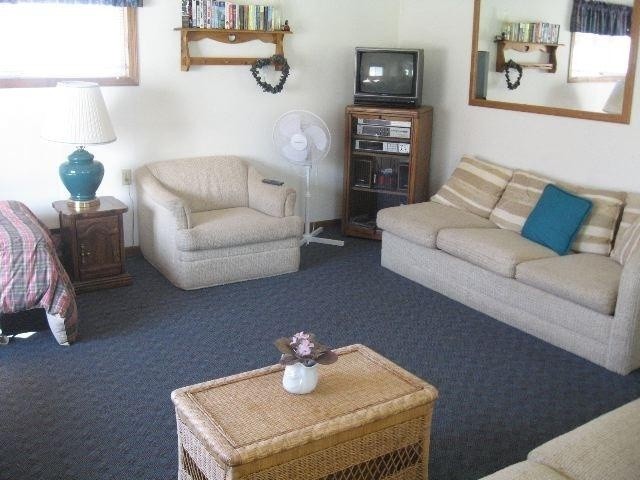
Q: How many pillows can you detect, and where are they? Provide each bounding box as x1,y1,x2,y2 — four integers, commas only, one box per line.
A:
564,187,627,256
429,154,514,219
488,168,558,235
521,183,593,256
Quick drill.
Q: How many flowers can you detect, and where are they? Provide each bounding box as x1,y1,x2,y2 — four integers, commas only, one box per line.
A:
274,330,338,366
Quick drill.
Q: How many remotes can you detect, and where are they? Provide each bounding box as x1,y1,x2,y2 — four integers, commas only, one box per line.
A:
261,178,285,187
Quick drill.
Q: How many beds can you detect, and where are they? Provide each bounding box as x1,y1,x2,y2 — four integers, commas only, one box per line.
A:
0,199,79,347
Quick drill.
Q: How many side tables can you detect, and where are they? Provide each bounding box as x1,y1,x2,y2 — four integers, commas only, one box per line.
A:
52,196,134,293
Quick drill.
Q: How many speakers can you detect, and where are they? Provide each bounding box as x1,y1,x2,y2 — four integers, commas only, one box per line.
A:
398,163,408,193
352,156,373,189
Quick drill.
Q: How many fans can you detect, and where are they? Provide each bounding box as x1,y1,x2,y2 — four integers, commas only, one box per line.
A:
272,110,344,247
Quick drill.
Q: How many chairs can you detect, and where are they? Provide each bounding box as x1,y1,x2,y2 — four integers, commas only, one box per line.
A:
134,155,304,291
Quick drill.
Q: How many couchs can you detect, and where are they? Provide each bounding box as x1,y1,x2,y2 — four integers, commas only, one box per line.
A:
480,396,640,480
376,155,640,376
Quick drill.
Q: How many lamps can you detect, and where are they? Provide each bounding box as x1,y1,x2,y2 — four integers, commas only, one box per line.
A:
47,80,117,205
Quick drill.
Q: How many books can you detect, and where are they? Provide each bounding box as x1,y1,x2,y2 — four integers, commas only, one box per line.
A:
191,0,281,31
503,21,560,43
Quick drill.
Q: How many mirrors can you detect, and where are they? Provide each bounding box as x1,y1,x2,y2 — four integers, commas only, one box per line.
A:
468,0,640,125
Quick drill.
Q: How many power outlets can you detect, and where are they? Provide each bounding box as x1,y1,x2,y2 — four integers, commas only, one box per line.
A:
122,169,133,186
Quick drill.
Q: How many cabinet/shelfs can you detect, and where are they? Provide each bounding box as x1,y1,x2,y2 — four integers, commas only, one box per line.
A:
341,105,434,238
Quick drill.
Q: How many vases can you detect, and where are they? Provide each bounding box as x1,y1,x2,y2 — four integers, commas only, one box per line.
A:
282,364,317,395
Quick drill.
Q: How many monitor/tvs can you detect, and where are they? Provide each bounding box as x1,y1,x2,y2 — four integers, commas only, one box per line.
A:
354,46,424,108
475,50,490,99
